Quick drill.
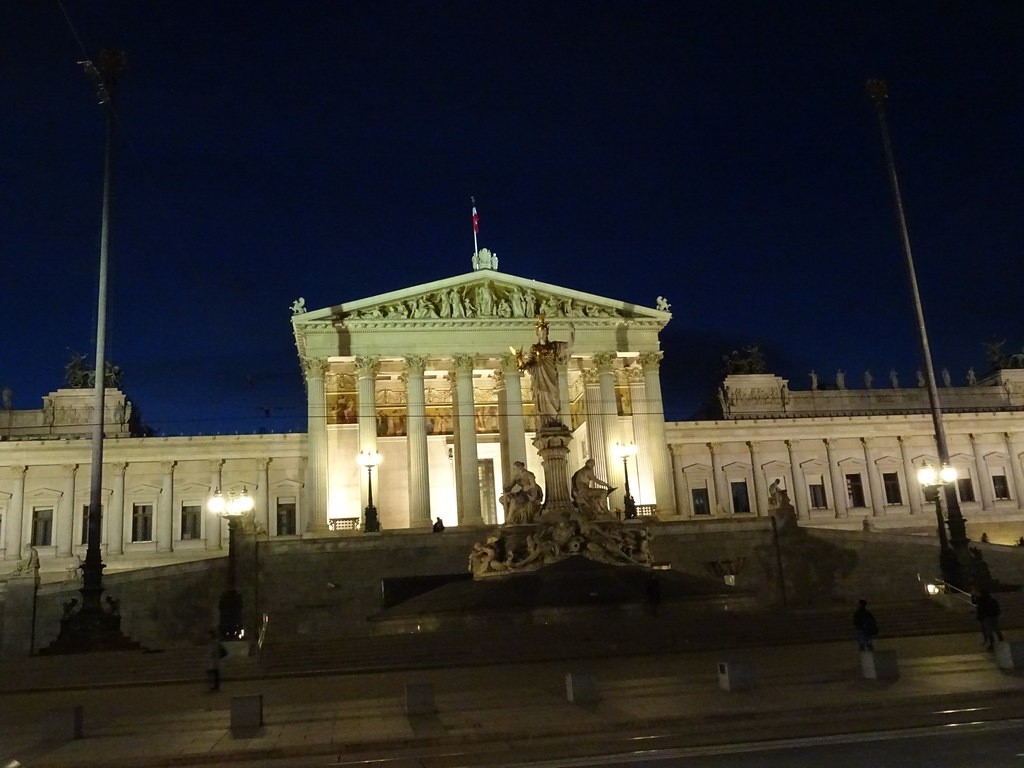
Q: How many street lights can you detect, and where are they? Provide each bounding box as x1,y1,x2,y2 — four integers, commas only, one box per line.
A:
356,447,382,534
915,456,965,592
206,485,254,691
613,441,639,521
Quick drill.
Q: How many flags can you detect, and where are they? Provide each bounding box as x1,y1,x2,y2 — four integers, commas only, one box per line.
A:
472,200,478,233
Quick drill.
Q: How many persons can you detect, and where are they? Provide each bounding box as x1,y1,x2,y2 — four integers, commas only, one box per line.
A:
206,630,220,690
432,517,445,533
853,600,876,651
971,588,1006,651
1015,537,1024,547
863,516,871,531
69,555,85,581
810,365,977,393
25,544,40,574
769,479,790,501
981,533,990,543
347,249,654,572
